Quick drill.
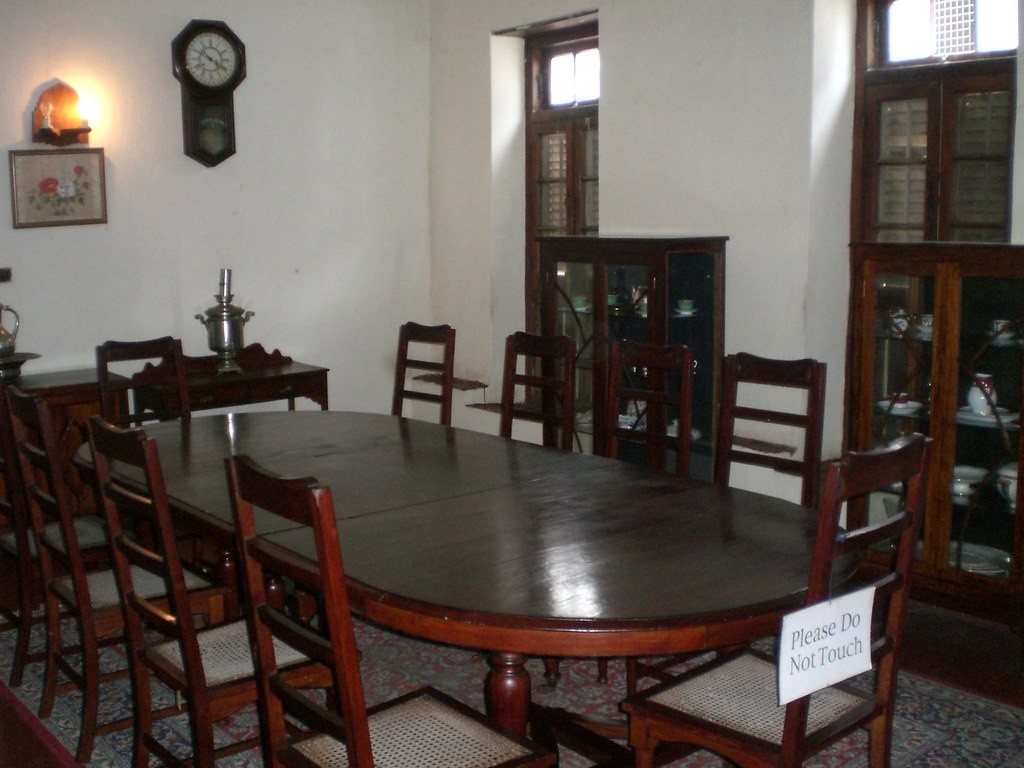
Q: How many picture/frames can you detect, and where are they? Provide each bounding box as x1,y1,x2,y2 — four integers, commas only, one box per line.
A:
9,148,107,228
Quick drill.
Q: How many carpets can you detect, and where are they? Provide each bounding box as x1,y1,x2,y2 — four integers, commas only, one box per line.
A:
0,600,1024,768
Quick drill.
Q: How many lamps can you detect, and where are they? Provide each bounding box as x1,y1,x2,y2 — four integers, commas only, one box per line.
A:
33,83,92,144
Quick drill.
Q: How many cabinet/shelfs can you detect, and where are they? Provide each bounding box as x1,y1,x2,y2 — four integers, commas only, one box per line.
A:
847,243,1024,629
535,236,729,486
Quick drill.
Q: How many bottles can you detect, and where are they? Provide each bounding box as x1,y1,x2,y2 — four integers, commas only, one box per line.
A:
968,374,997,417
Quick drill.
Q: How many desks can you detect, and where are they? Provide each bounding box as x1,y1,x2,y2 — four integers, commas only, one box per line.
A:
76,413,862,768
0,369,134,570
134,353,329,427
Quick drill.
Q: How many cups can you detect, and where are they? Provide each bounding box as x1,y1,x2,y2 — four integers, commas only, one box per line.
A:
994,321,1012,339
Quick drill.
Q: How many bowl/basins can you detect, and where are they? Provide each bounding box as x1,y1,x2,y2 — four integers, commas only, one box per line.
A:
953,466,987,495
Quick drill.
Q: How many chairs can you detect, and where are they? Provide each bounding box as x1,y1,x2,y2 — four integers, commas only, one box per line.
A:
97,337,192,429
4,380,229,764
501,332,575,450
0,406,144,688
628,353,827,696
598,339,693,682
86,417,361,768
622,430,927,768
223,454,557,768
392,321,456,426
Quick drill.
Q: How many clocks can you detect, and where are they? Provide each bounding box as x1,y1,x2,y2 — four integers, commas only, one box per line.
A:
172,19,247,166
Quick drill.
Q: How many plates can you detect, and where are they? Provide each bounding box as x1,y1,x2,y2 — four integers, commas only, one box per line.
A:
957,405,1011,423
878,401,922,414
916,540,1011,576
